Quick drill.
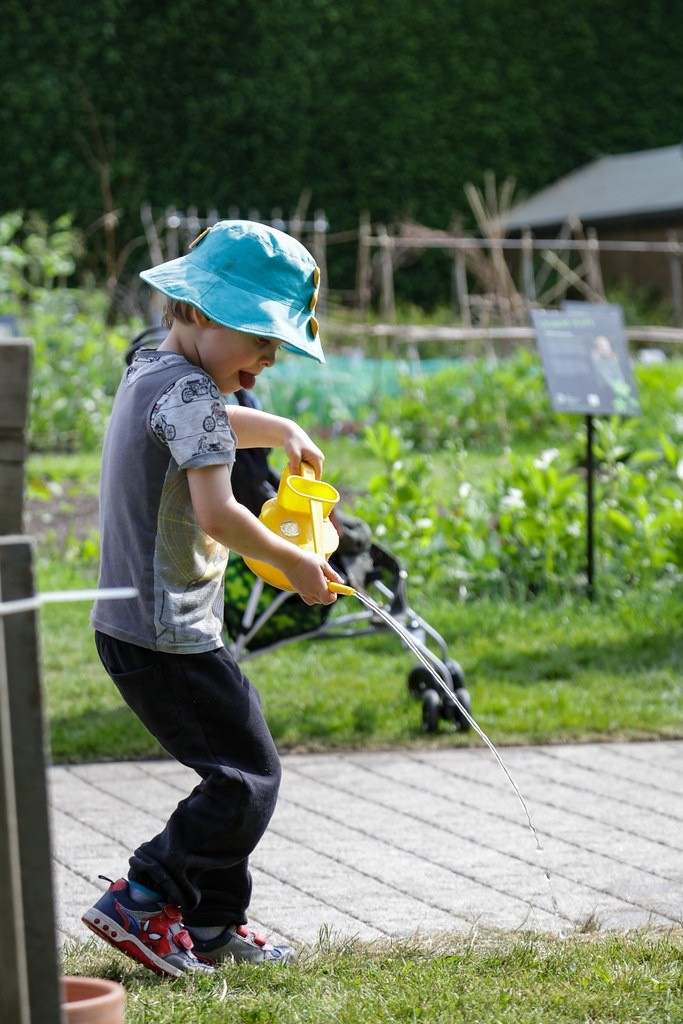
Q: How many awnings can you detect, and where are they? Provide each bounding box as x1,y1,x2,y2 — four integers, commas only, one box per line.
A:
475,143,683,257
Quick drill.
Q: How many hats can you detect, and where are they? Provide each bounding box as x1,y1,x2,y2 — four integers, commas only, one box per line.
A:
138,220,327,364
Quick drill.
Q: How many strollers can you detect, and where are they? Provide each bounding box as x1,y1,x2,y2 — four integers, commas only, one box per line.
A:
223,390,472,736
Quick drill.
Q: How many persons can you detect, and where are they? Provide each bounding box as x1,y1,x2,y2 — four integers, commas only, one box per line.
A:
81,220,345,982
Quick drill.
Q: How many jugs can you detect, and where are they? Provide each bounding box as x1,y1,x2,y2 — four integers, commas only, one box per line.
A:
241,460,357,596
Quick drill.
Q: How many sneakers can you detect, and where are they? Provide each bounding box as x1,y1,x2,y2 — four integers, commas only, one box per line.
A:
82,874,215,979
184,923,296,970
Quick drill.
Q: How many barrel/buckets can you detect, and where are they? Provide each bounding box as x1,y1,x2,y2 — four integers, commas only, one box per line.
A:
61,976,129,1024
